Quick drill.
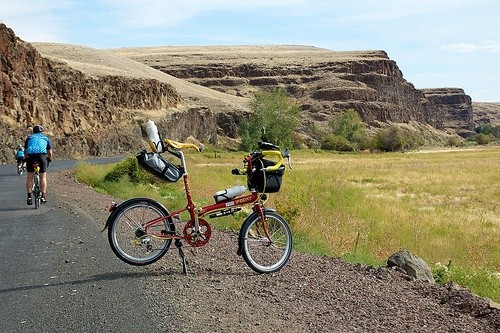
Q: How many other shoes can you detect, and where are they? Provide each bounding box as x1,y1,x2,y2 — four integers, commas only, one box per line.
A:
41,197,47,203
27,199,32,205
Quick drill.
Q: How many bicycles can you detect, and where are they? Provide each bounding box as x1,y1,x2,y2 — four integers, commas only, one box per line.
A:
24,157,53,209
14,157,26,177
100,140,294,276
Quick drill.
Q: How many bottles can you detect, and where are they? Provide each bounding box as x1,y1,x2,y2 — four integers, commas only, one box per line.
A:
215,185,248,201
146,119,163,154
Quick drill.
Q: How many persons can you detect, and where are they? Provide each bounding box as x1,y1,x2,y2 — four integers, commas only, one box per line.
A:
24,125,52,206
16,144,25,173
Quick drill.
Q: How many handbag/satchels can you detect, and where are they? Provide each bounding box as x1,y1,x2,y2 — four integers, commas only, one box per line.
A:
136,151,181,183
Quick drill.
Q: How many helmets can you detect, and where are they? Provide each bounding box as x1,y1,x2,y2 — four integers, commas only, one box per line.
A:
19,147,23,151
33,125,42,132
